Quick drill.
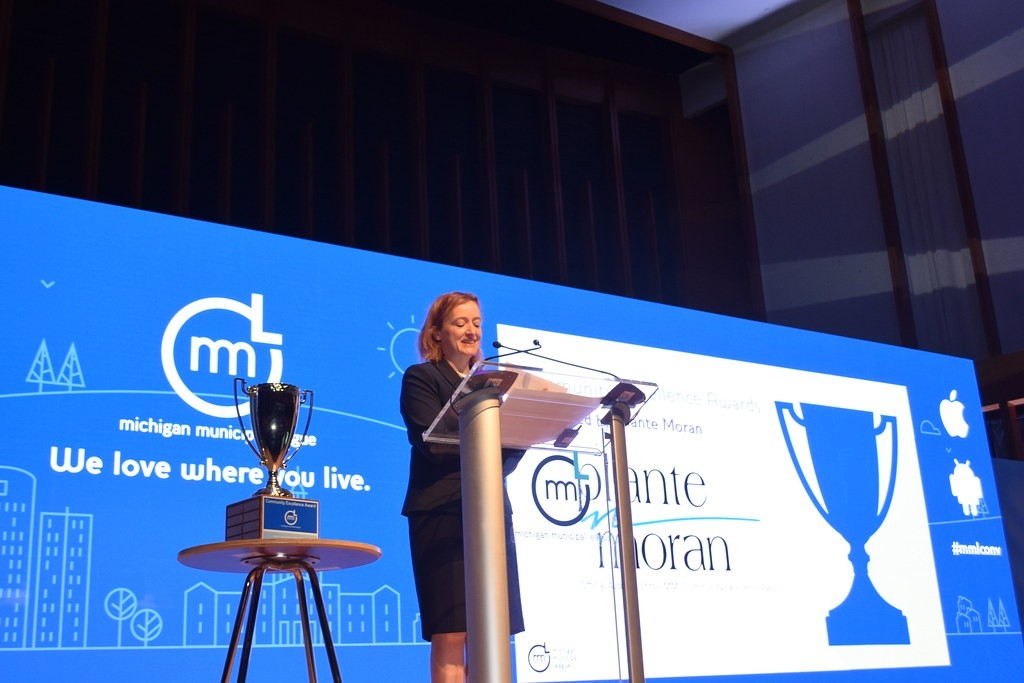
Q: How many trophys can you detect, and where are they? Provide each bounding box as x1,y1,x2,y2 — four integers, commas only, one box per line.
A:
233,376,314,500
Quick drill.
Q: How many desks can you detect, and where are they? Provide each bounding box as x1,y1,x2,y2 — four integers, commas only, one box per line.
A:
177,539,382,683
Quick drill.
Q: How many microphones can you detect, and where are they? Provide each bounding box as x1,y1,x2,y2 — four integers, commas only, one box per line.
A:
479,339,620,378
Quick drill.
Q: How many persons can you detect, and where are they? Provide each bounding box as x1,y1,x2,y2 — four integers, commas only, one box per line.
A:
397,289,527,682
